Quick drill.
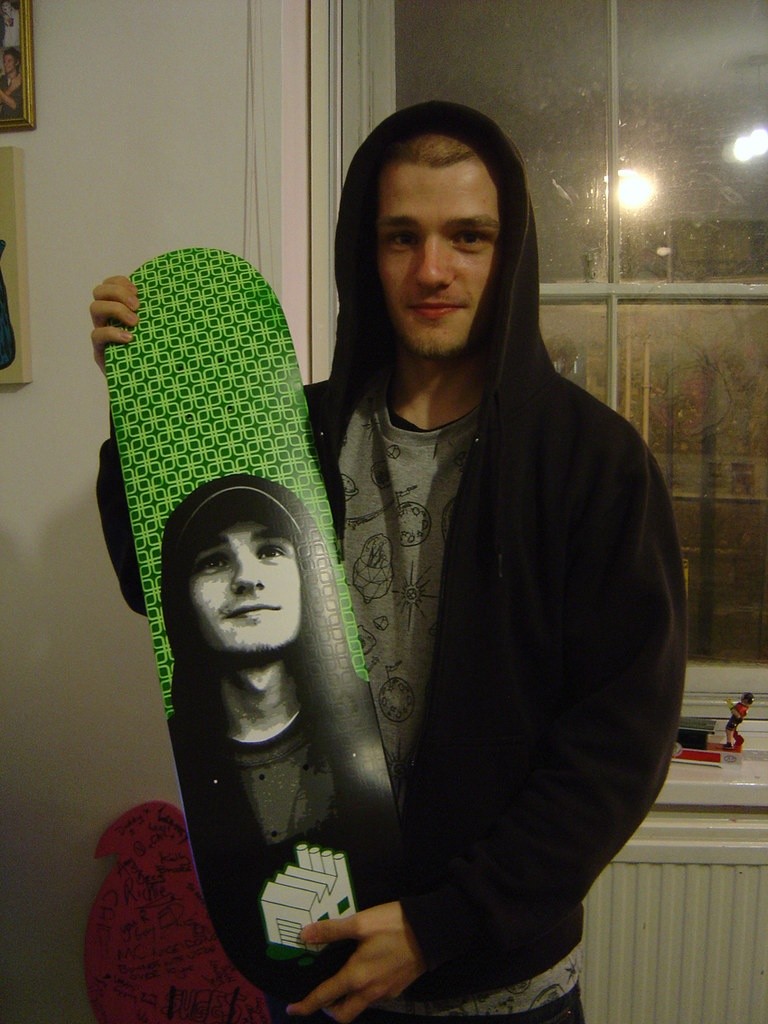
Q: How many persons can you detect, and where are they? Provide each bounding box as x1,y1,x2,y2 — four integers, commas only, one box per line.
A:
1,47,24,118
154,475,383,942
91,102,689,1023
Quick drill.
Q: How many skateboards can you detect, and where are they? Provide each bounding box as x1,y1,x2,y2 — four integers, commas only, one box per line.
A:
103,248,408,1002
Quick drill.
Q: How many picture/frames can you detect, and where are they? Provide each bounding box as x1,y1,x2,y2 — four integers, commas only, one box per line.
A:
0,0,36,132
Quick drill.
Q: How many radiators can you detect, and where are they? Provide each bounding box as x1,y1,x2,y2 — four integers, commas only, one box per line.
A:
580,814,768,1024
